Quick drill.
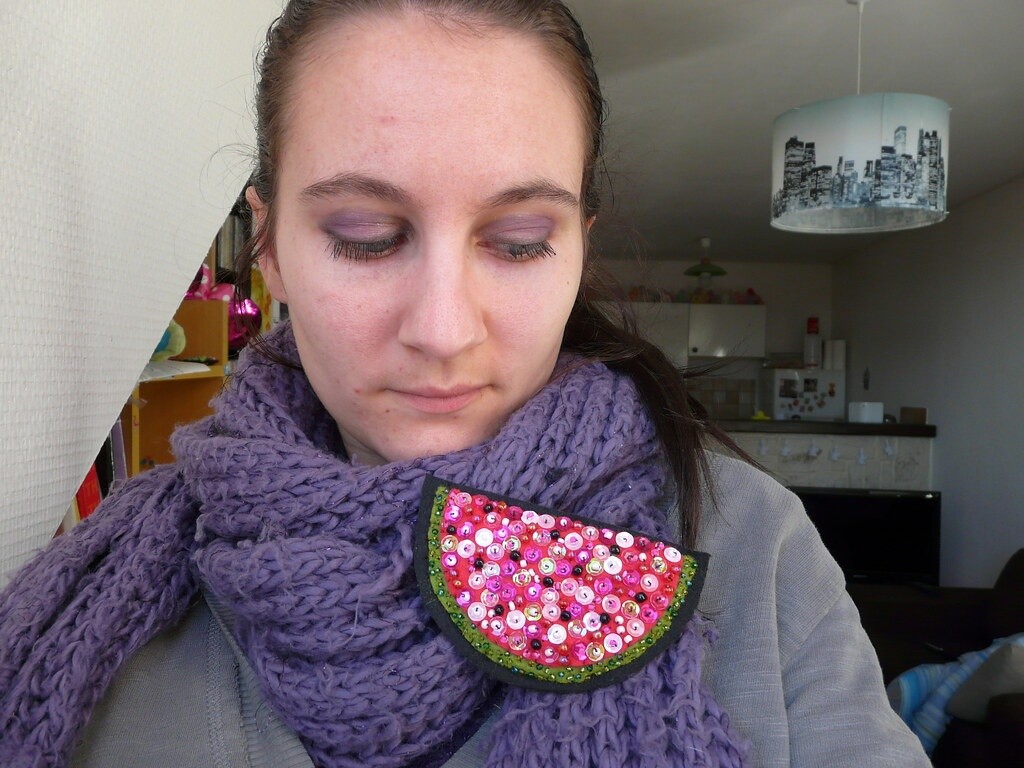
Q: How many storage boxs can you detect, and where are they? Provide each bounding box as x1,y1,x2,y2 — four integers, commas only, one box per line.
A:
760,353,846,421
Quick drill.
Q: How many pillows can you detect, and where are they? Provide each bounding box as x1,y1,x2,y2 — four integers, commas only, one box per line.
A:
944,644,1024,722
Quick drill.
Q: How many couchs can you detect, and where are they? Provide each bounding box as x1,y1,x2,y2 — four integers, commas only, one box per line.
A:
846,548,1024,768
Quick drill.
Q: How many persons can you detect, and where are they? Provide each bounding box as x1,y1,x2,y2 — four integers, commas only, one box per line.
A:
0,0,937,768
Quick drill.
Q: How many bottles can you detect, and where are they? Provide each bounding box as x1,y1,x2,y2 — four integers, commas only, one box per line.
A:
804,316,822,370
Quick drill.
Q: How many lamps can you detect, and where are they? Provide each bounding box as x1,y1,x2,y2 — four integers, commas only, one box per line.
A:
770,0,953,234
684,237,728,287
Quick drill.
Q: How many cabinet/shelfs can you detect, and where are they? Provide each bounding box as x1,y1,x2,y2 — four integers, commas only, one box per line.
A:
119,241,229,479
688,304,766,358
591,301,688,366
